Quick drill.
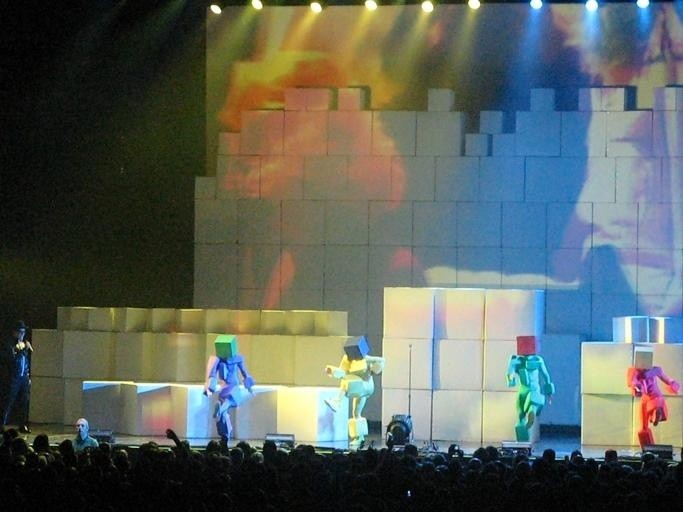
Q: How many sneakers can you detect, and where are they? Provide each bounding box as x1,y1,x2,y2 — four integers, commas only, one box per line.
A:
651,408,662,427
325,400,340,413
213,401,222,422
525,411,534,431
349,438,365,446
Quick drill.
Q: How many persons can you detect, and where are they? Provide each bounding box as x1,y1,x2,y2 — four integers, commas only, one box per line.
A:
624,344,680,454
504,332,557,442
320,332,390,455
0,317,35,434
201,332,255,443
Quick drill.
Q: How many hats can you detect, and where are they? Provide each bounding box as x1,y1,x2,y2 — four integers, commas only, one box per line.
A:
10,320,28,331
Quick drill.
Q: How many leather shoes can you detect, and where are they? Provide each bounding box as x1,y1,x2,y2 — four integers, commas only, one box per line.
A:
19,424,31,433
